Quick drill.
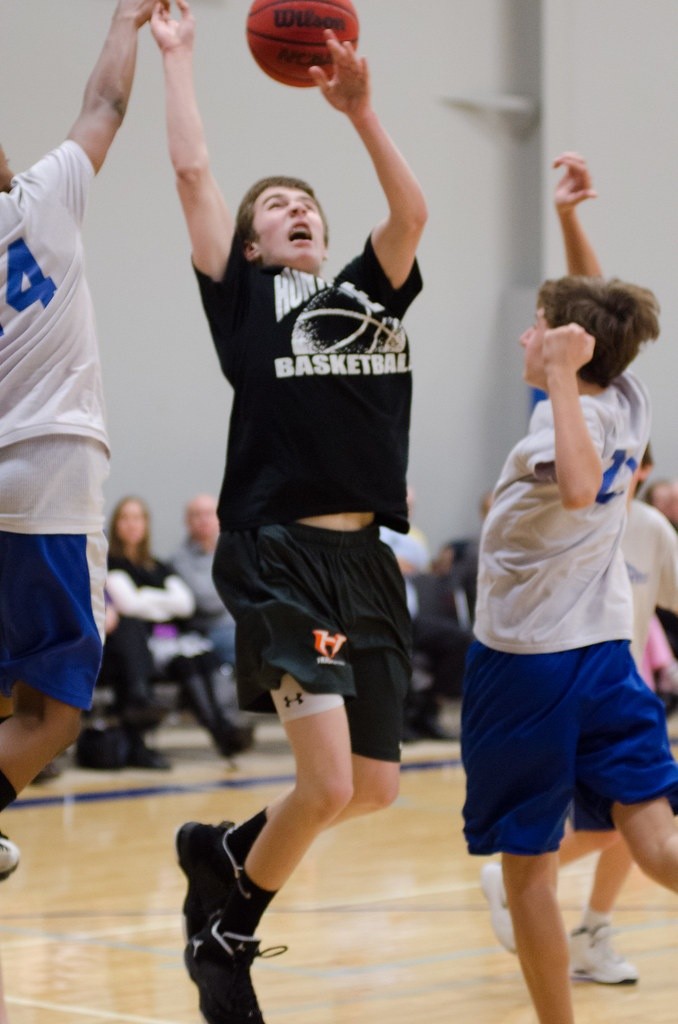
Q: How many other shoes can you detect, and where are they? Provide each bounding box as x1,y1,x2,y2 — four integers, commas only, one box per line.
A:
0,835,20,882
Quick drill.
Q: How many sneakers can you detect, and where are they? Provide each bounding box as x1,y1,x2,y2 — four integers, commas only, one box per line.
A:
481,862,516,955
176,820,244,941
569,924,638,985
184,909,288,1024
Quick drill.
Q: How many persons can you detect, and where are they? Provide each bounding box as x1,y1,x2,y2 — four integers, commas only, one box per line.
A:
0,1,162,885
456,153,678,1024
0,494,258,785
376,485,494,740
144,0,431,1024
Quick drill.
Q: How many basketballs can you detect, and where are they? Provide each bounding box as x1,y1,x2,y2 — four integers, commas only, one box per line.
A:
246,0,358,88
